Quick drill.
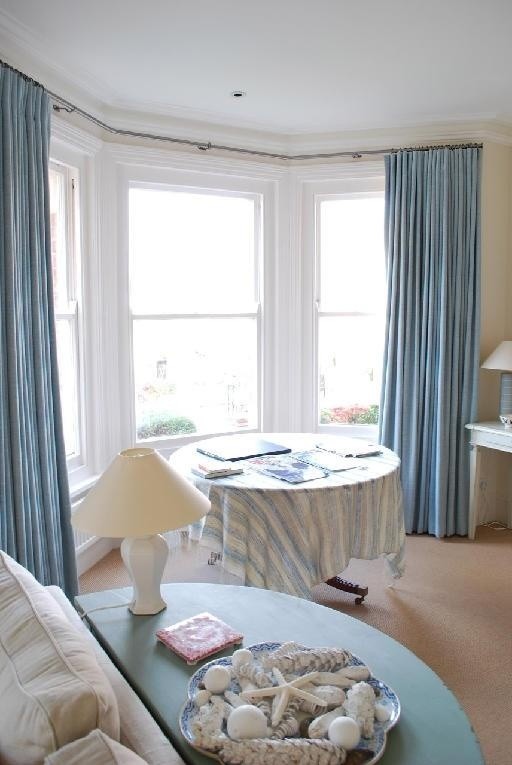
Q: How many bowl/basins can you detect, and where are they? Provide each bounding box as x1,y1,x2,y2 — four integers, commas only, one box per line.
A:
499,415,512,427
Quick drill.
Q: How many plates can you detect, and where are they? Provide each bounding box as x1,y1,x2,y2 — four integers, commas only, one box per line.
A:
180,642,401,764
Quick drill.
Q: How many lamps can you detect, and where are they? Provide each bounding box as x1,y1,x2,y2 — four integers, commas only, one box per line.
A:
71,448,212,616
481,340,512,416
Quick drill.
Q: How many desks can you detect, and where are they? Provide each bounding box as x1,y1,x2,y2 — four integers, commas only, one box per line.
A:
170,433,406,605
75,582,484,765
464,421,512,540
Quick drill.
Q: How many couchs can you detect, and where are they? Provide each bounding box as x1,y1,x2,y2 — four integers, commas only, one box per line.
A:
45,585,186,765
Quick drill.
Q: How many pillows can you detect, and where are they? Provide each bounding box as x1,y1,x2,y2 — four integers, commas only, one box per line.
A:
0,550,148,765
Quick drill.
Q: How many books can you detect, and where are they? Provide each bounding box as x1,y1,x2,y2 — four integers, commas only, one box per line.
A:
246,449,358,485
158,613,241,664
317,434,382,459
192,462,243,480
196,434,292,461
